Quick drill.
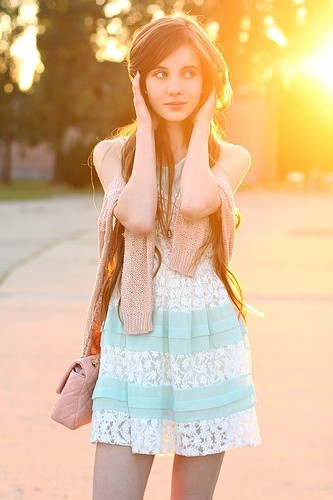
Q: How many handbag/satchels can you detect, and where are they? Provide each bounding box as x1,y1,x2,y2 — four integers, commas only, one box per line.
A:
49,352,103,432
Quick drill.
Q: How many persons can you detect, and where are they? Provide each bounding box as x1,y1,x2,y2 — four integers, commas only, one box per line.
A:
88,15,262,500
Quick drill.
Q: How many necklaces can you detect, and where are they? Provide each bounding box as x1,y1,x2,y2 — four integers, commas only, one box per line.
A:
154,187,180,240
